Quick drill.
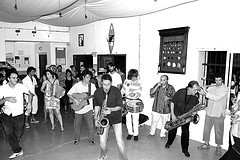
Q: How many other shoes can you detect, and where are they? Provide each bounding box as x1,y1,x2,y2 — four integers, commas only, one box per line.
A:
73,139,79,145
161,137,166,141
60,126,65,134
51,125,56,133
165,143,170,149
147,134,155,139
9,149,24,158
197,144,211,149
127,134,133,140
182,150,191,157
216,146,223,156
25,122,31,131
134,136,139,142
89,140,95,146
30,119,40,123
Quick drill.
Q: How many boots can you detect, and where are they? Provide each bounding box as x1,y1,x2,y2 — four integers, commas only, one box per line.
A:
97,145,108,160
118,146,129,160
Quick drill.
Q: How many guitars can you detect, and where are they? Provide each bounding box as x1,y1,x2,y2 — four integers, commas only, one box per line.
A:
68,92,95,111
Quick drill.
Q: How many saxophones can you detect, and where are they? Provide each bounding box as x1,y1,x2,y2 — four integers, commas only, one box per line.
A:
95,89,109,136
164,92,207,131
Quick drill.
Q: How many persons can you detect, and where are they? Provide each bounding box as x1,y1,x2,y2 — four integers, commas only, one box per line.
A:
198,74,228,154
147,75,175,141
0,63,142,160
229,80,240,147
165,81,200,157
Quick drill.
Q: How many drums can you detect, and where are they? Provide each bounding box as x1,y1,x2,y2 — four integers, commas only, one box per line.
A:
126,99,145,113
